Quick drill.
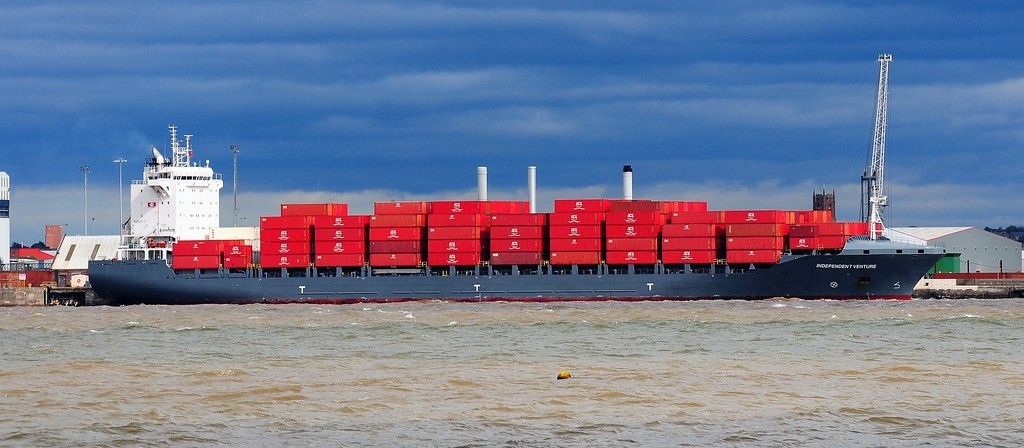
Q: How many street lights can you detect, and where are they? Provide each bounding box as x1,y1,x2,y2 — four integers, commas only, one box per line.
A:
79,166,92,237
112,157,128,236
229,144,240,227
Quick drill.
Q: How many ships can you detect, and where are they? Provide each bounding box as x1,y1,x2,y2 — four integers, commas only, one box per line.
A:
88,236,964,307
